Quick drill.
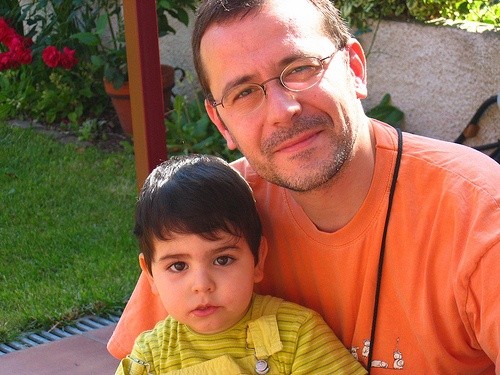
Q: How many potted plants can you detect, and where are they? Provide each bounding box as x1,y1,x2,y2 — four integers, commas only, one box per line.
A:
103,0,197,134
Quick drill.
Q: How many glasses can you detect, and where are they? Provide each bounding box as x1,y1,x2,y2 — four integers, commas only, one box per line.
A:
207,42,345,117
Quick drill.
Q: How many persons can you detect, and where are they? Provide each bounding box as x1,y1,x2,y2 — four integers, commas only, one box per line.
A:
113,154,369,375
105,0,500,375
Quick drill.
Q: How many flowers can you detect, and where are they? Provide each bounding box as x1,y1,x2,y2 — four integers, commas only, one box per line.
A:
43,46,79,70
0,18,33,72
